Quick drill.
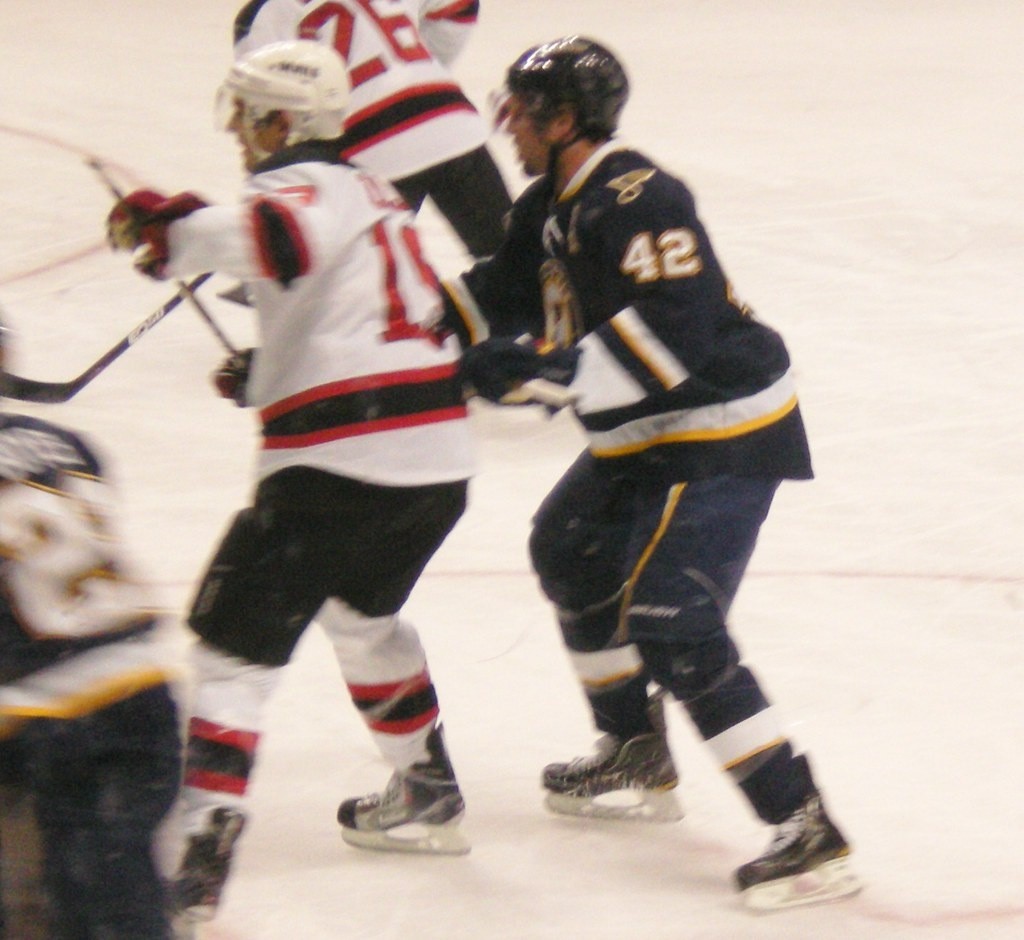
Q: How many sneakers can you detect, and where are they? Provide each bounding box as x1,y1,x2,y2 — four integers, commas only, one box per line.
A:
337,722,471,854
539,684,684,825
733,755,860,912
149,800,247,940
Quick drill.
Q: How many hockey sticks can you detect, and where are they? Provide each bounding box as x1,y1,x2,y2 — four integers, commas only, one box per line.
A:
88,152,247,368
0,270,215,406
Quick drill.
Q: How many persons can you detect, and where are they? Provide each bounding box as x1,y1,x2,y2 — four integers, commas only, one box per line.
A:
233,0,513,258
108,40,474,921
439,36,865,916
0,314,183,940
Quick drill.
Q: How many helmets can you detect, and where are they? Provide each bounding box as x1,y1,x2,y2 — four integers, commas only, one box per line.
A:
507,36,630,149
224,40,353,147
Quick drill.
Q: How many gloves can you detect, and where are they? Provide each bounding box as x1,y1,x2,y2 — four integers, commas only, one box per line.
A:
212,348,255,407
106,187,209,282
455,330,584,418
